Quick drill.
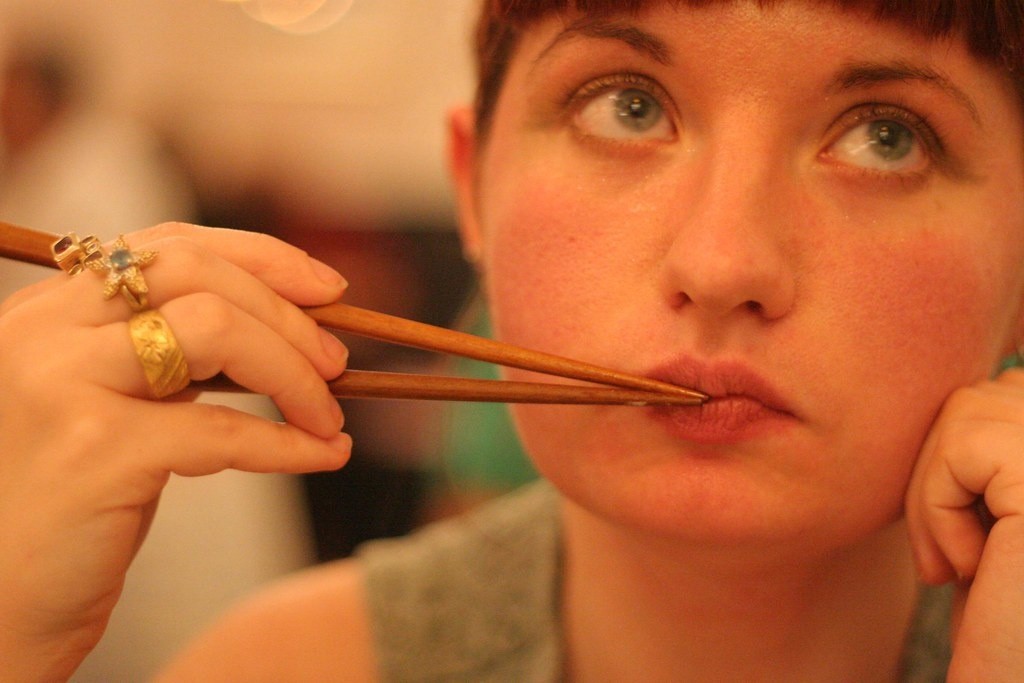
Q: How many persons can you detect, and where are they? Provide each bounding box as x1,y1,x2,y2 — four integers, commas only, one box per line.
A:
0,1,1024,683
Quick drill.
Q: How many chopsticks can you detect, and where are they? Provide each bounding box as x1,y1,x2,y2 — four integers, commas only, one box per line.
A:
0,221,709,406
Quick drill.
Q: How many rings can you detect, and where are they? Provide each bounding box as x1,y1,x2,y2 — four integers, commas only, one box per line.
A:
85,232,160,311
50,230,107,275
128,311,190,397
